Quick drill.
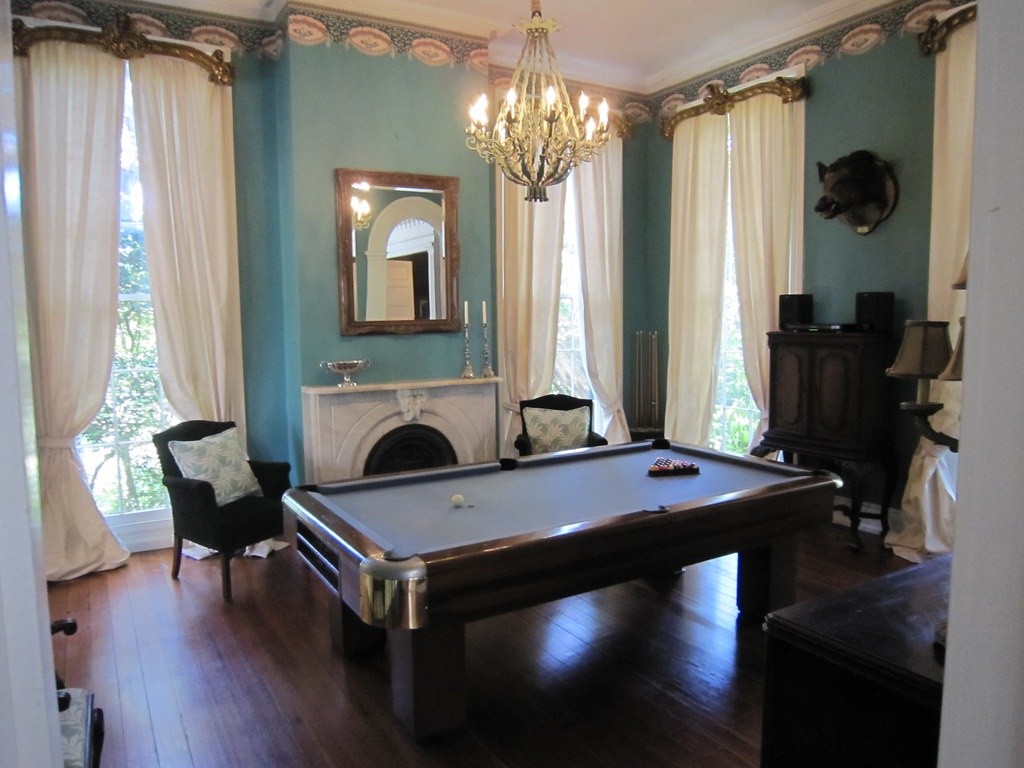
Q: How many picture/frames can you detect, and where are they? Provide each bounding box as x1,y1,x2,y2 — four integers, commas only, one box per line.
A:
334,168,461,337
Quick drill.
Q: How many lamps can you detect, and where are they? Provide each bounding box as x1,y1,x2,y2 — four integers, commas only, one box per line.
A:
886,249,969,451
464,0,612,202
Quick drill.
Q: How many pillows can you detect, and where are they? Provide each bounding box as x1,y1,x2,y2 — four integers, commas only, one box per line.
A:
523,406,591,456
168,427,263,509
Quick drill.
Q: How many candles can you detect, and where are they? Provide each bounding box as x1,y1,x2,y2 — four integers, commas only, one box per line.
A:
481,301,487,324
464,300,469,324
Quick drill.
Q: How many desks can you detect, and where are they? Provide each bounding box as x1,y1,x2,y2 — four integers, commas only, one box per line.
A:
281,438,846,749
758,553,952,767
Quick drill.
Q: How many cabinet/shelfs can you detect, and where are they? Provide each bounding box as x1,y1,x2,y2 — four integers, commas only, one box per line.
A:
760,330,921,537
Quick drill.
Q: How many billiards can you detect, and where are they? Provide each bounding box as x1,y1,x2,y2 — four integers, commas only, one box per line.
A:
648,456,699,472
451,494,465,508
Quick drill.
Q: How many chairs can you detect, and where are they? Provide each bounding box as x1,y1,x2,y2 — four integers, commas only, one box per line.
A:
153,421,293,601
50,617,104,768
514,394,609,456
